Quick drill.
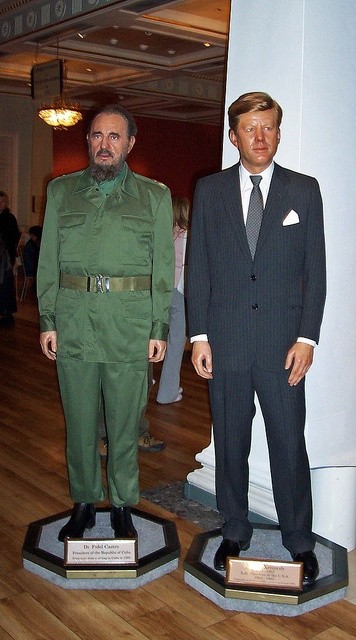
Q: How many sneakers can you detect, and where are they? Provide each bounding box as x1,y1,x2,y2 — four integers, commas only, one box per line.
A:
97,438,108,464
138,430,164,451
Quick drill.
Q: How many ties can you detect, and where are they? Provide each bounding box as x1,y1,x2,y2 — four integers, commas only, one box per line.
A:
245,176,263,261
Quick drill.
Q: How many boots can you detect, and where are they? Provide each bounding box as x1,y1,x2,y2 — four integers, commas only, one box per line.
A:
110,504,138,538
58,502,96,542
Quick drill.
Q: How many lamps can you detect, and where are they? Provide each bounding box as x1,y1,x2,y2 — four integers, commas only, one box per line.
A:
37,61,85,131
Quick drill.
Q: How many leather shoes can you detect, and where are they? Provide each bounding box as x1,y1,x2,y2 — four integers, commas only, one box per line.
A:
290,550,319,582
178,387,184,394
156,394,183,405
214,539,250,571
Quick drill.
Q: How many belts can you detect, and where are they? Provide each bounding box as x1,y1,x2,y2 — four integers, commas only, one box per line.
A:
60,271,151,294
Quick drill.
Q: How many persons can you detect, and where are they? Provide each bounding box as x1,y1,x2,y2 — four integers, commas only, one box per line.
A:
37,107,174,542
156,193,192,404
0,192,20,331
184,91,327,585
90,361,165,457
25,226,39,281
